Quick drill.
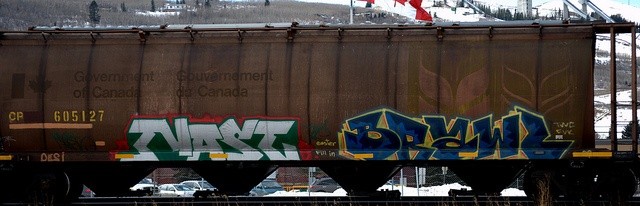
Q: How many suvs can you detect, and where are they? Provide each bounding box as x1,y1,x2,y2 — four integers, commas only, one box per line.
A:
308,178,338,193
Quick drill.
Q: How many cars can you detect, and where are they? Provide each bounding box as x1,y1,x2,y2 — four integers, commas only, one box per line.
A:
159,183,195,197
129,179,158,192
250,180,284,197
180,180,216,190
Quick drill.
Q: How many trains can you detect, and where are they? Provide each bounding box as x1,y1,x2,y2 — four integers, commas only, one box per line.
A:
0,17,637,205
